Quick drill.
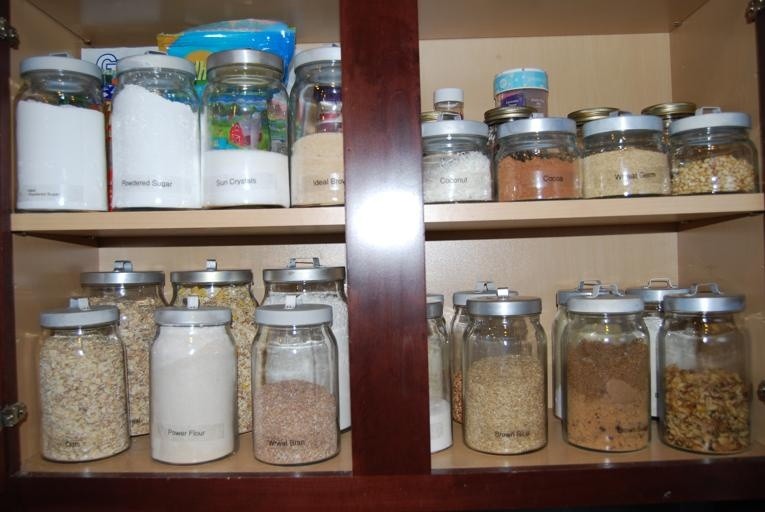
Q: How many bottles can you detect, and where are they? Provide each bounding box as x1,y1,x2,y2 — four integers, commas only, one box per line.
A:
201,49,292,208
423,65,760,205
290,45,346,208
110,53,202,209
16,56,108,211
30,258,755,463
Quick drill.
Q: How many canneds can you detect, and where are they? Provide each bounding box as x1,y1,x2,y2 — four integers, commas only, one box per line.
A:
422,103,759,203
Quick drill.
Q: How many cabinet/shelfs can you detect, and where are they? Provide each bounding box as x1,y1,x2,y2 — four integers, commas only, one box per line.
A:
0,1,765,512
